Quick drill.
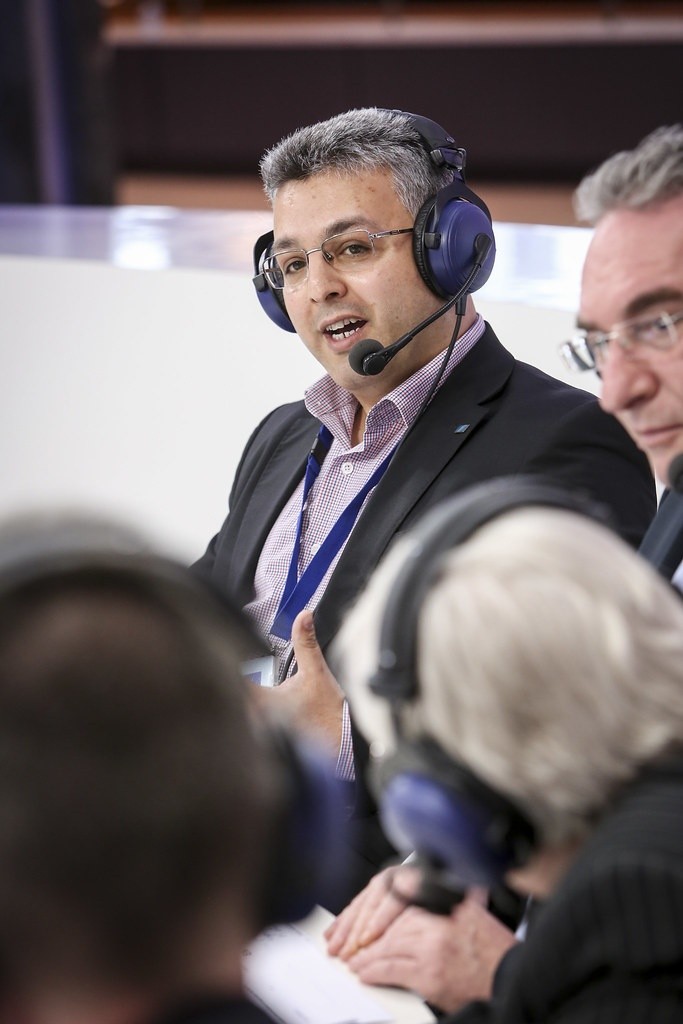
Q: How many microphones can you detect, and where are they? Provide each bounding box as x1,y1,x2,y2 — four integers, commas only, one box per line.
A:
350,264,482,376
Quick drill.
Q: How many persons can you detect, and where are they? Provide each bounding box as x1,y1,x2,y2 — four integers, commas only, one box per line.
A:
325,125,683,1022
0,509,334,1022
340,476,683,1023
188,106,657,916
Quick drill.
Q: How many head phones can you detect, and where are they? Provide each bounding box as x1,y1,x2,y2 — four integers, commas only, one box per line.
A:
370,482,628,892
0,535,361,926
253,111,495,333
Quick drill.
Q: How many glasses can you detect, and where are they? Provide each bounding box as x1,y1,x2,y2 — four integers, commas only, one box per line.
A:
260,227,413,291
559,309,683,372
